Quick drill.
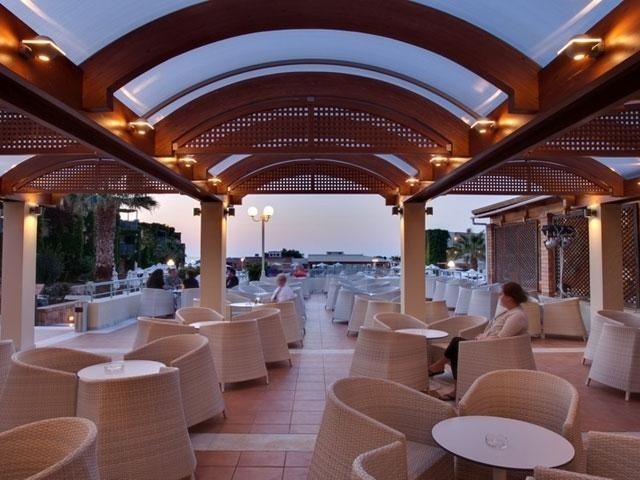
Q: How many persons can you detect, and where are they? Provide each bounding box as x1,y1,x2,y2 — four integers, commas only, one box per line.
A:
271,274,295,304
226,270,239,288
147,268,199,309
427,282,529,401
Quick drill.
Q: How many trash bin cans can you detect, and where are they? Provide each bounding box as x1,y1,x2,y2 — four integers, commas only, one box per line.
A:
73,301,88,333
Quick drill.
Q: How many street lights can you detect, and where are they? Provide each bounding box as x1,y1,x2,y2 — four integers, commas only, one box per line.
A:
248,205,273,281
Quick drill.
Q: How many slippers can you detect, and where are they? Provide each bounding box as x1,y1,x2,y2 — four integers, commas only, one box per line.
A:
428,367,445,377
439,393,456,401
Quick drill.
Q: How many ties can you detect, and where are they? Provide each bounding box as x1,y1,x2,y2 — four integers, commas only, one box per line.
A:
274,288,281,299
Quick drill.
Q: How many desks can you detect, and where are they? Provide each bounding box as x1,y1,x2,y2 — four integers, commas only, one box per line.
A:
431,414,575,480
74,358,167,381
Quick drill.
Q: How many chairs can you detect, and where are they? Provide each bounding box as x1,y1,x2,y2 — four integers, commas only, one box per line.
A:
458,370,585,478
1,347,114,429
75,367,198,479
0,415,100,478
1,271,320,393
122,333,227,427
349,441,408,479
534,430,640,479
321,268,639,404
306,376,458,478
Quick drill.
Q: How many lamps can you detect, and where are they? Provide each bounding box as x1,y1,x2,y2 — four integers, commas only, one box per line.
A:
393,207,403,215
224,208,235,216
582,207,597,218
405,176,419,187
29,206,43,215
180,155,197,168
208,175,223,186
556,33,603,64
426,207,433,215
470,119,498,135
127,117,155,135
429,154,448,169
194,208,201,215
22,36,65,63
